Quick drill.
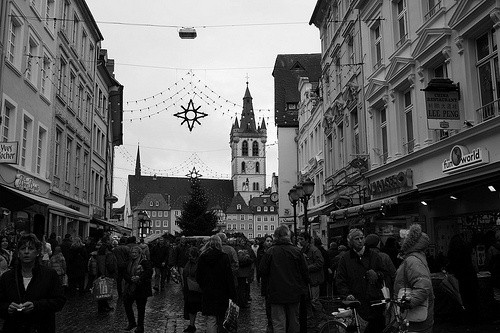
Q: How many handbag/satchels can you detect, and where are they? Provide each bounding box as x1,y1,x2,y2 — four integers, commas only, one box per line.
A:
223,298,239,332
396,288,429,322
94,275,113,299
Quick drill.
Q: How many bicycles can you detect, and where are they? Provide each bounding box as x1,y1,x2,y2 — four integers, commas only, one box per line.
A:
318,297,417,333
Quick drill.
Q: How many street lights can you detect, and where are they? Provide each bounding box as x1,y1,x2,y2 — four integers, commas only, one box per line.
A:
295,176,315,233
288,184,298,238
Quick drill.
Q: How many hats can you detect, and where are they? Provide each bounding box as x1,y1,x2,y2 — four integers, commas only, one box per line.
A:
330,242,338,250
366,233,379,247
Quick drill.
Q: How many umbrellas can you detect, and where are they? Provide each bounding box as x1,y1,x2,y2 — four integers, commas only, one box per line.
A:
439,267,465,310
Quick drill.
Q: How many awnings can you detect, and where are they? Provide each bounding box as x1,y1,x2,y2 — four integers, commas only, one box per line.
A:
330,190,418,222
0,183,132,231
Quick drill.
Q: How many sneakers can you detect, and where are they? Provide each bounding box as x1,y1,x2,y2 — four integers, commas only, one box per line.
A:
308,321,328,329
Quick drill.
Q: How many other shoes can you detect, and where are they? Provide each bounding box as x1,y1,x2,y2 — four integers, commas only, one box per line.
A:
124,324,145,333
182,325,196,333
98,307,114,314
153,285,165,291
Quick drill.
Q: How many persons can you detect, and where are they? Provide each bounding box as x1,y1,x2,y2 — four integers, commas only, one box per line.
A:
152,224,500,333
0,231,153,333
